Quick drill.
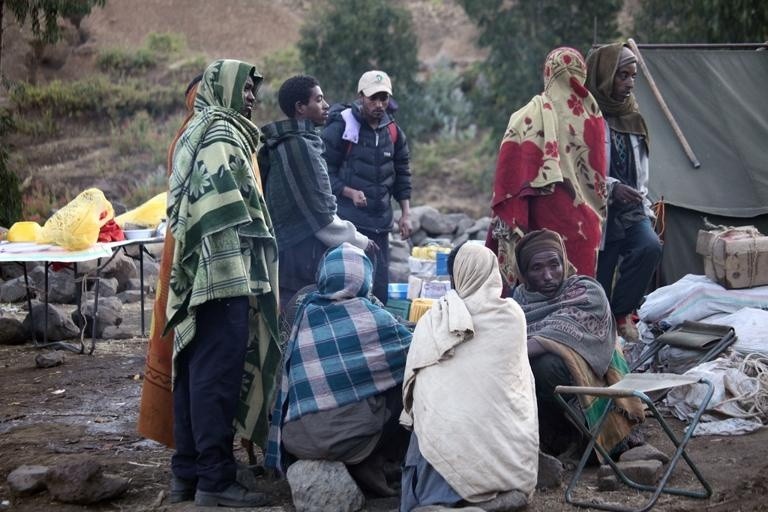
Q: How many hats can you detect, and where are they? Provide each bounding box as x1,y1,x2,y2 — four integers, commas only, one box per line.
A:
357,70,393,97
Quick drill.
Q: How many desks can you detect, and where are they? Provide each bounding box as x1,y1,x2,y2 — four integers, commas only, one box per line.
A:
0,239,166,356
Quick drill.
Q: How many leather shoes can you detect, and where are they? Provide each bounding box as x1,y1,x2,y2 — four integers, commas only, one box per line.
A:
169,475,199,503
194,483,273,507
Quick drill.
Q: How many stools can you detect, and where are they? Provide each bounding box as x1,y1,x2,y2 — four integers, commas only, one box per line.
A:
552,373,716,512
625,319,738,410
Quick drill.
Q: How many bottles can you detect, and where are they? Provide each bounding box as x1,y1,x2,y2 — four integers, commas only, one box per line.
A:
158,217,167,238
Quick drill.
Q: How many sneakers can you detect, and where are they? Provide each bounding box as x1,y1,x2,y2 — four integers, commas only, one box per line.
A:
615,312,639,343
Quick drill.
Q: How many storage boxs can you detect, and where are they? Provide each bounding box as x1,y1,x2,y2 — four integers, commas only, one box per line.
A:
383,245,453,322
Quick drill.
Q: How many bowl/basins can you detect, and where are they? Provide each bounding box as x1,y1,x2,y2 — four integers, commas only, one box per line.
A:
6,220,44,242
387,283,409,299
121,229,157,241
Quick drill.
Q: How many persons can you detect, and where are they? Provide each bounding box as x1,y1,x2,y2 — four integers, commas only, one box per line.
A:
398,241,539,512
159,59,282,506
318,68,412,307
259,74,381,314
139,74,263,452
484,47,610,300
514,230,646,483
585,43,662,342
263,243,415,497
